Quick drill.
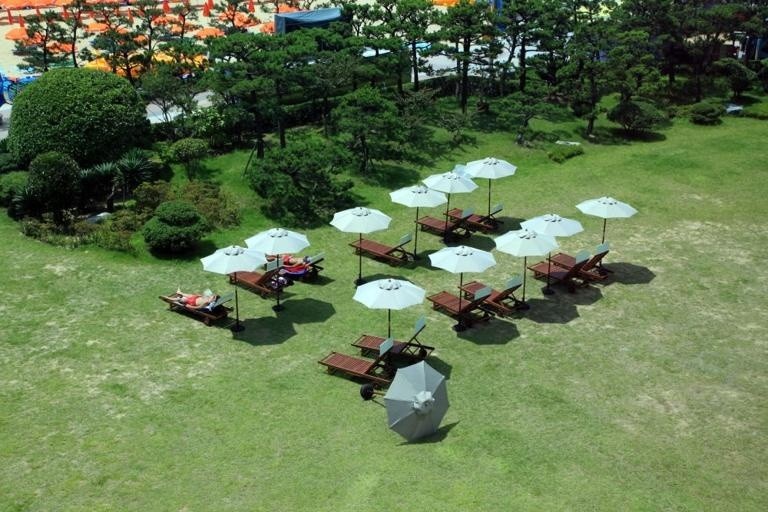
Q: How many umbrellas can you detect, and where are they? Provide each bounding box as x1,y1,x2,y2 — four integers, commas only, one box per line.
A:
353,278,426,360
368,360,450,441
330,206,393,280
199,243,266,324
241,227,312,305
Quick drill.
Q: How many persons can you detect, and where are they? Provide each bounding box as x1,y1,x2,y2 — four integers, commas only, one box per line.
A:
159,287,221,312
270,253,309,268
